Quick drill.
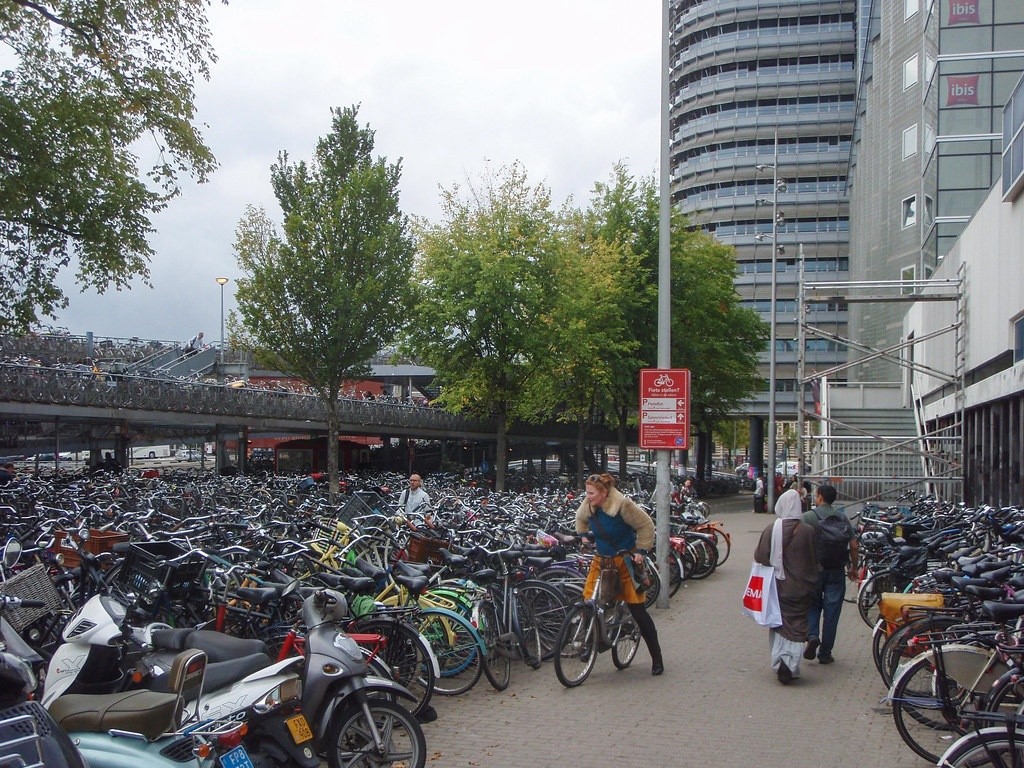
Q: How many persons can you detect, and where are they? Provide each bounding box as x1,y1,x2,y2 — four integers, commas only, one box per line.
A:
183,333,208,352
575,472,665,675
396,474,433,524
753,471,860,686
362,391,375,402
273,380,287,397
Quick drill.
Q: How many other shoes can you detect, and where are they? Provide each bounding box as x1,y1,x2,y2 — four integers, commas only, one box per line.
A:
778,663,799,683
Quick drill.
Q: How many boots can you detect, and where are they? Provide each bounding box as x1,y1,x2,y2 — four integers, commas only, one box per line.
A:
580,612,594,661
643,630,665,675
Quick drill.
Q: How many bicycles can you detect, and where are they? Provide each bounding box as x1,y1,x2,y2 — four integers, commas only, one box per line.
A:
0,335,459,428
0,465,733,730
844,489,1024,768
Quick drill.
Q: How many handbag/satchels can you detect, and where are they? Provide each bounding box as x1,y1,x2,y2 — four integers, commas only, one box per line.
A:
623,554,655,596
742,562,783,629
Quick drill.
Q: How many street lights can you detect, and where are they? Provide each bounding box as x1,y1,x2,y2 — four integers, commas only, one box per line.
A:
755,163,788,512
216,278,229,384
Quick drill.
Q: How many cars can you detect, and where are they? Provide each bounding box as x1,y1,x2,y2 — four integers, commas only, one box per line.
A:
735,463,750,474
776,462,798,476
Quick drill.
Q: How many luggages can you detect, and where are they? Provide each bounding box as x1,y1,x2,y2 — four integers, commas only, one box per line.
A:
754,494,766,513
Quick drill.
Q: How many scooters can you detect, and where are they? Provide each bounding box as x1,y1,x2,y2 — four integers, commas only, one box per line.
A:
47,647,254,768
0,537,90,768
40,574,320,768
130,578,428,768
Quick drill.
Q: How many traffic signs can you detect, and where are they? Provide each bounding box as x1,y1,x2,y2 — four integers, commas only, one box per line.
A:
642,398,687,424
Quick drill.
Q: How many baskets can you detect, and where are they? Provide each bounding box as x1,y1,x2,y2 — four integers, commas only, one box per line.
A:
118,541,204,598
339,491,396,533
312,518,353,553
54,528,129,569
409,535,450,565
0,564,63,632
299,477,315,490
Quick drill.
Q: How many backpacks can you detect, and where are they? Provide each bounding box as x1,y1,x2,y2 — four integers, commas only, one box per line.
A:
811,507,851,571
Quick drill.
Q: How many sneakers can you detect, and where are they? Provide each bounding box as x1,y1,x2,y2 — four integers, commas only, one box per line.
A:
803,636,835,665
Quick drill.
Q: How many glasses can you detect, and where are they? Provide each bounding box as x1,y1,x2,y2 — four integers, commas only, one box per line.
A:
589,476,607,489
